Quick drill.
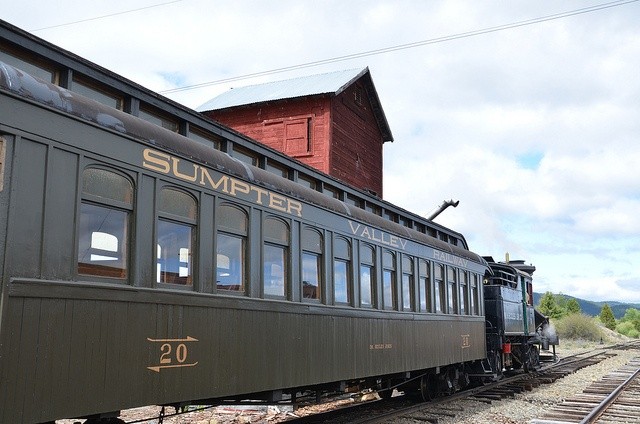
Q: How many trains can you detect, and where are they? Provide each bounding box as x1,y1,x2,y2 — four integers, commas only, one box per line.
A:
0,16,551,424
